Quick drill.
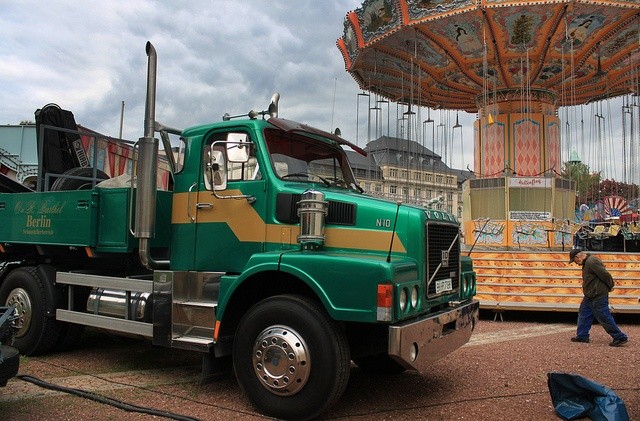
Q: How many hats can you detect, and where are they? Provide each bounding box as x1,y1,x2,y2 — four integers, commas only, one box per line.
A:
569,249,581,264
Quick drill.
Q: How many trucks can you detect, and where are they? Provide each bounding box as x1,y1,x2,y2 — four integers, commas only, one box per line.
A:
1,40,480,421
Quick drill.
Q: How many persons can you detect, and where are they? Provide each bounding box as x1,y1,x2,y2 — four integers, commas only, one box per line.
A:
569,249,629,347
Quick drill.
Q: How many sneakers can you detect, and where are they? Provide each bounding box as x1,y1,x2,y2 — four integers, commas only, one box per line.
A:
609,337,627,346
571,337,589,343
